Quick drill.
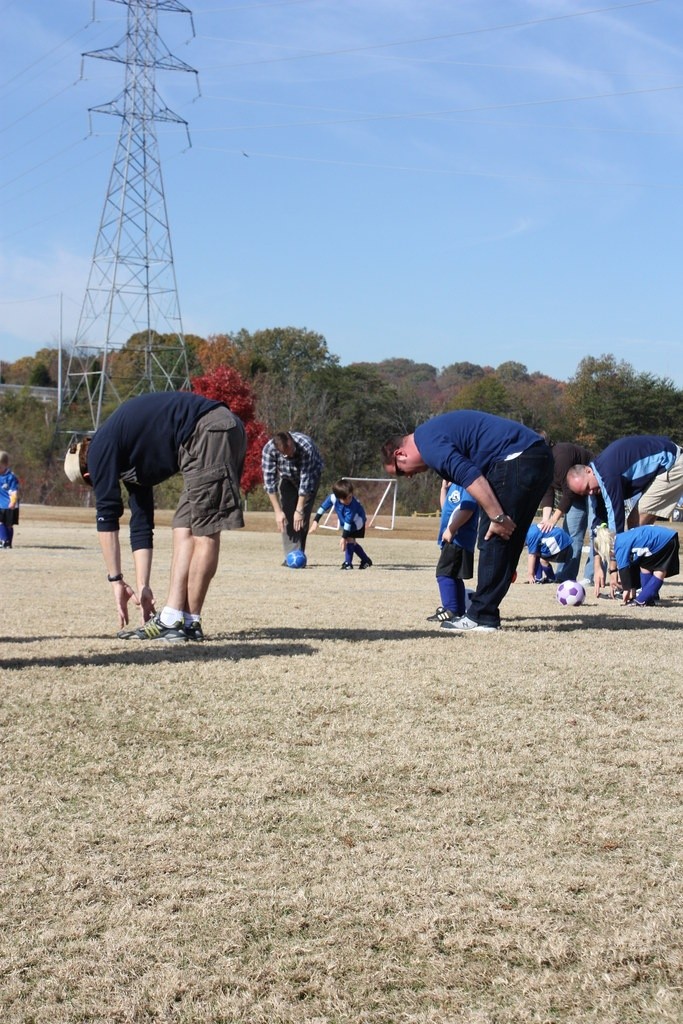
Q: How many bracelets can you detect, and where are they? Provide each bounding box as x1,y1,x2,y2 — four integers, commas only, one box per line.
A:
108,574,123,582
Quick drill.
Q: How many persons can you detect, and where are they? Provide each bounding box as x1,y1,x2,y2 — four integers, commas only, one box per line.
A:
533,428,608,586
593,525,680,607
262,430,323,567
309,480,373,570
0,451,20,549
426,480,480,623
566,435,683,600
381,410,555,632
523,522,574,584
64,392,247,643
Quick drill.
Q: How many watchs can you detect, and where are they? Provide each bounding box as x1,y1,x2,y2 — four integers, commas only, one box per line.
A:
490,513,506,523
607,568,618,574
296,509,304,515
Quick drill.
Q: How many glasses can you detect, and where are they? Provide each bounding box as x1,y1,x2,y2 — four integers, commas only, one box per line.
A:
394,457,406,478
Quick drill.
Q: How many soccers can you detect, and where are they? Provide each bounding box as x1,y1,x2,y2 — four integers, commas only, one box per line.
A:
288,550,308,568
556,579,587,607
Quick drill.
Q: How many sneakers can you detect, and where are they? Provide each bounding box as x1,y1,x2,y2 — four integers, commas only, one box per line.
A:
185,616,204,642
578,578,594,587
636,593,660,600
117,611,187,642
426,607,465,622
626,599,655,607
435,607,445,614
359,559,372,569
441,614,498,632
340,561,353,569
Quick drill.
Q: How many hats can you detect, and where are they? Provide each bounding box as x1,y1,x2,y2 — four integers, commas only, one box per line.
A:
63,441,93,489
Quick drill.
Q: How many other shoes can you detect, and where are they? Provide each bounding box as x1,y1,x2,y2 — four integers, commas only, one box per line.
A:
535,576,556,583
281,560,288,566
0,540,11,548
